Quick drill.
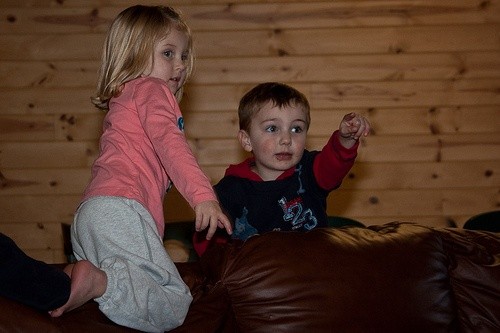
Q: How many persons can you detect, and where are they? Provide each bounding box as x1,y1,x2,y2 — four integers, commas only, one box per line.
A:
0,232,77,316
49,4,233,333
192,81,372,261
